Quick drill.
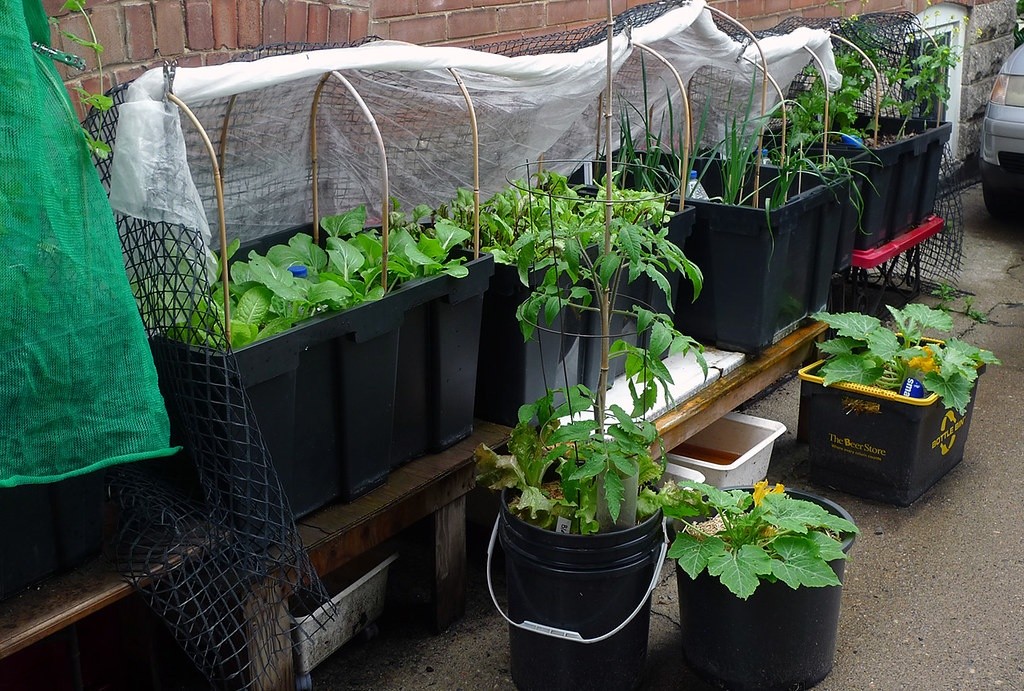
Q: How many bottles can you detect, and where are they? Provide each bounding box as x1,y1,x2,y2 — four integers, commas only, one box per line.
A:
757,148,770,165
685,170,709,201
287,266,307,277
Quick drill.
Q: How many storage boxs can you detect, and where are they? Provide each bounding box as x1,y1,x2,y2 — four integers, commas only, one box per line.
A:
292,551,404,674
656,413,788,490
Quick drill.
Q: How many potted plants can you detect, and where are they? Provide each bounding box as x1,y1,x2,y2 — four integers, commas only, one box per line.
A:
51,0,1003,691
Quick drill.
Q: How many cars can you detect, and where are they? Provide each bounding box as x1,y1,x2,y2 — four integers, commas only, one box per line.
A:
977,42,1024,220
